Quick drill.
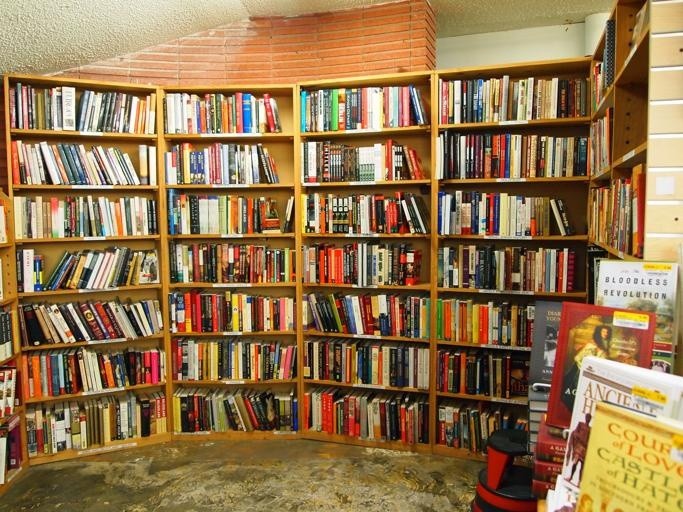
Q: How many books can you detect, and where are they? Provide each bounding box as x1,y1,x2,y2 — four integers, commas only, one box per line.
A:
165,91,298,435
528,261,683,510
591,109,612,176
589,163,645,260
1,83,167,484
437,75,591,454
301,84,430,445
591,20,615,105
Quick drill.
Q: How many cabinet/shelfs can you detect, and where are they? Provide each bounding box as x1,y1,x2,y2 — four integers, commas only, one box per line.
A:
161,84,300,441
431,55,596,469
2,73,32,497
7,75,172,468
589,1,649,294
295,70,433,456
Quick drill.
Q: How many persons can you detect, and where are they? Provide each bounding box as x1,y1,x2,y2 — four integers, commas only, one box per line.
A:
588,324,612,360
566,413,592,487
